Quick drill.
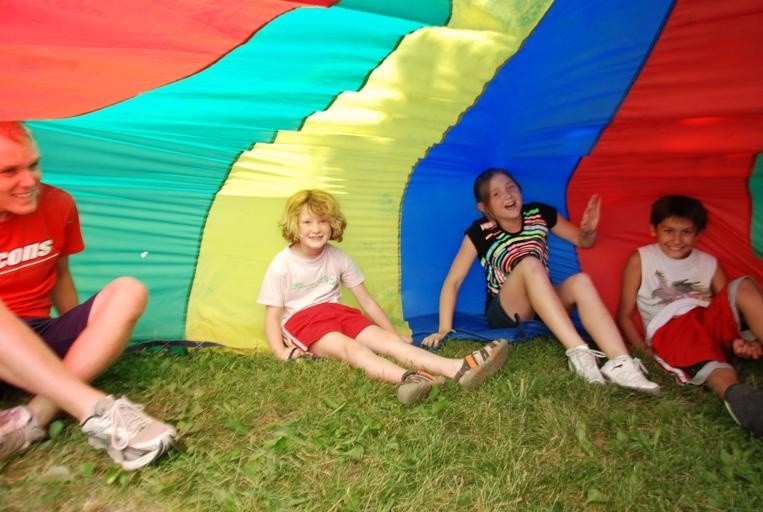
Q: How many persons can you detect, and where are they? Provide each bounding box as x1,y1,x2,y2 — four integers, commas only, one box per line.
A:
616,193,763,437
730,335,761,363
254,189,510,408
420,167,663,396
0,120,180,473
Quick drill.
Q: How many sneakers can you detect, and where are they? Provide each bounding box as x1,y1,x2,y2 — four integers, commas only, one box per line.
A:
78,394,178,471
396,369,445,405
565,345,660,395
0,406,47,471
454,338,509,386
